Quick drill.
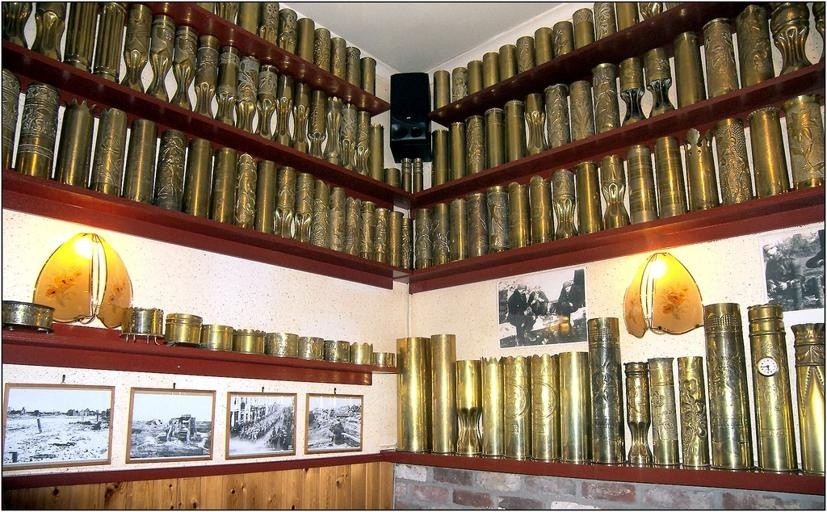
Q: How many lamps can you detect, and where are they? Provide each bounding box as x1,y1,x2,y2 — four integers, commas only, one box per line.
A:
624,252,704,338
33,233,134,329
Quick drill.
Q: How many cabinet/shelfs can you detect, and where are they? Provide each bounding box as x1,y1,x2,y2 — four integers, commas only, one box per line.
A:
394,2,825,295
1,1,413,290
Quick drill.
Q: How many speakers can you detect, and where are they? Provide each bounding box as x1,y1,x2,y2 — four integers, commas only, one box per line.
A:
390,72,431,163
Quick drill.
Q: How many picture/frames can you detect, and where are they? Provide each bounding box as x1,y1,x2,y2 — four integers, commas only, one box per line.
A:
758,221,825,312
495,264,588,351
225,392,297,461
125,388,216,465
304,393,364,454
2,382,116,471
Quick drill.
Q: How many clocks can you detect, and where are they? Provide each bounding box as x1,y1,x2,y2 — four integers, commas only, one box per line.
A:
756,356,779,377
